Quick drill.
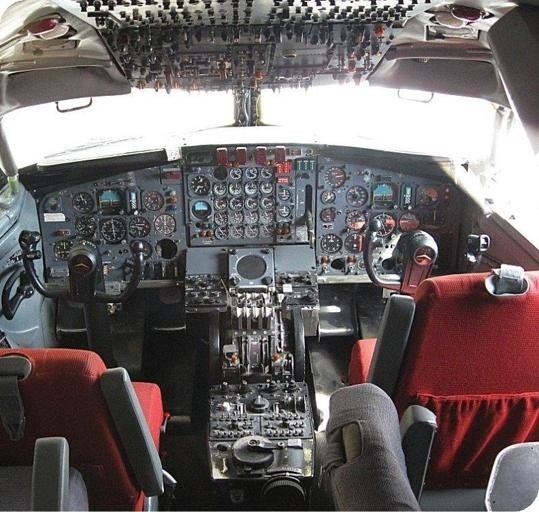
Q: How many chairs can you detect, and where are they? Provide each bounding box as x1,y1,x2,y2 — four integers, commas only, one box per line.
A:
347,271,539,489
0,347,191,512
311,381,539,512
0,435,89,511
